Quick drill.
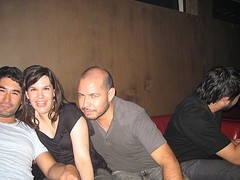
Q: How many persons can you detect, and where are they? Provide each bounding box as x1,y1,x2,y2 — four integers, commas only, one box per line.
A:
163,65,240,180
15,64,114,180
0,64,82,180
76,66,184,180
